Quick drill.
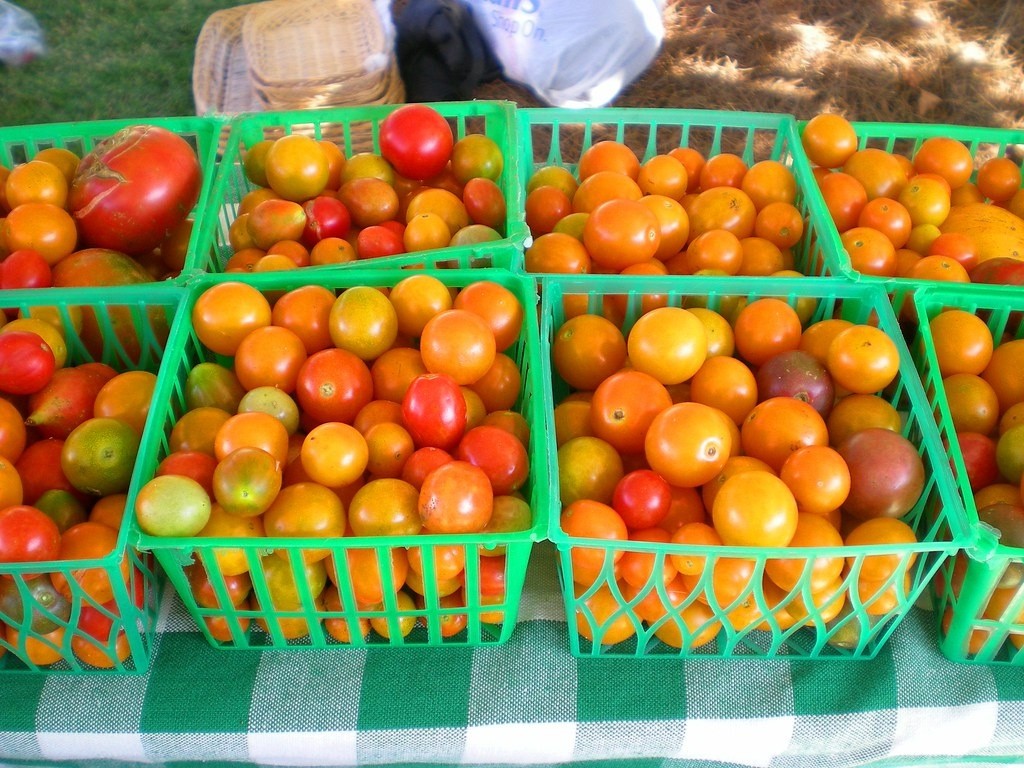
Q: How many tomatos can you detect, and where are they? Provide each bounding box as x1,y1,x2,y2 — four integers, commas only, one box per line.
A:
525,113,1024,657
0,105,531,667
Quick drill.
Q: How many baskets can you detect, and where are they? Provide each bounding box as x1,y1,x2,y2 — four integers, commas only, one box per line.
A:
0,288,189,678
914,287,1024,665
542,275,979,661
0,101,1023,293
128,267,550,648
192,1,412,154
